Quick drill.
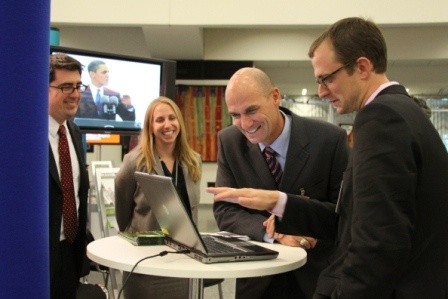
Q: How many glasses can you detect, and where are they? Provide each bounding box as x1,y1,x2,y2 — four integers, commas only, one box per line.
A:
317,55,368,89
49,83,88,94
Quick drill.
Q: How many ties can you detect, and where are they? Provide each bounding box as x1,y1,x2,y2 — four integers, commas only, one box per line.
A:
264,146,284,191
96,89,100,105
57,125,78,244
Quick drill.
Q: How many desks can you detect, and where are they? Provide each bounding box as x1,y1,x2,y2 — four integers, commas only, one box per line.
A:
86,235,307,299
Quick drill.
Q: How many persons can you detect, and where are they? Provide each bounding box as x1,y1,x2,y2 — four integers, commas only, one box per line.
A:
205,68,350,299
48,54,89,299
114,97,202,299
75,60,135,121
309,18,448,299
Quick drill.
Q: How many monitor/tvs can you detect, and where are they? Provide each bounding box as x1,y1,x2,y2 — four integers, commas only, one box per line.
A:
50,45,176,135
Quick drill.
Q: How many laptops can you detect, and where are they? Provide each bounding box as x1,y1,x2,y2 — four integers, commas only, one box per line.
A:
134,171,279,264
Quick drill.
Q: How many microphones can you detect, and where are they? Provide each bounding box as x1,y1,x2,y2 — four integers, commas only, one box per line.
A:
100,95,119,114
